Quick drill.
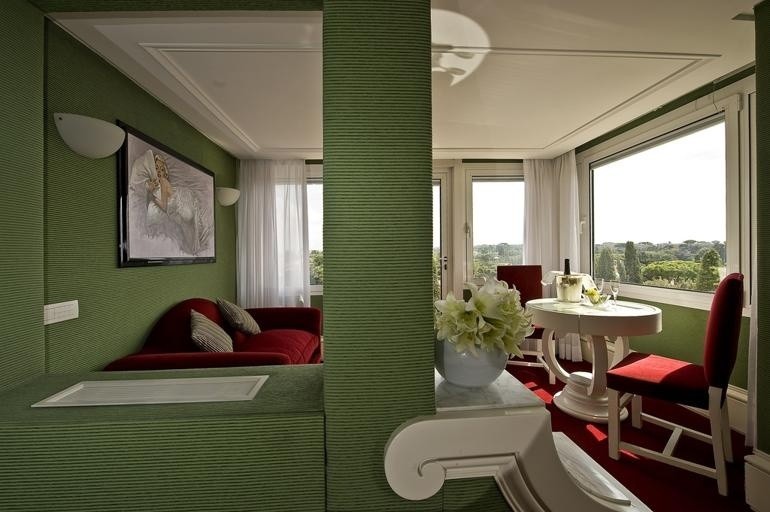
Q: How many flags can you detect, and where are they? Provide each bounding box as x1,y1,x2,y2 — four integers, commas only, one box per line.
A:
432,330,509,387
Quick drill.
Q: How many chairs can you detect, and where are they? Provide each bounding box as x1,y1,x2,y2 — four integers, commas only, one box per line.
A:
486,265,557,385
605,273,743,496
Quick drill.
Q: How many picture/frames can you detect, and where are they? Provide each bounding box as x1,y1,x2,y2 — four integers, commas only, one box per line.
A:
115,119,215,269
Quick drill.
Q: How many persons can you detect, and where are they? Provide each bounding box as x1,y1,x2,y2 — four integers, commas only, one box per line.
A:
144,154,197,227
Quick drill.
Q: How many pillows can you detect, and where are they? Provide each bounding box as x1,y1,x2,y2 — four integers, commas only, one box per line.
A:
191,297,262,353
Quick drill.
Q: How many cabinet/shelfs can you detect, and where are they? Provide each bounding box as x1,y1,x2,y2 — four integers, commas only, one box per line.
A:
563,259,571,276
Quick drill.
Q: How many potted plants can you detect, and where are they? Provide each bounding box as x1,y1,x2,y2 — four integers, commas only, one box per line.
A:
115,119,215,269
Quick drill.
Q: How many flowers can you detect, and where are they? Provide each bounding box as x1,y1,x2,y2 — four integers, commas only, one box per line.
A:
431,274,536,359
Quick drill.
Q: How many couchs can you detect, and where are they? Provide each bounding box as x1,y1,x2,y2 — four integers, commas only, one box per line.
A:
105,297,322,371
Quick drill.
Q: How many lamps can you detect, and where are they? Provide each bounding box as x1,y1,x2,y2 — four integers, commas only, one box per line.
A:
218,186,241,206
54,113,125,159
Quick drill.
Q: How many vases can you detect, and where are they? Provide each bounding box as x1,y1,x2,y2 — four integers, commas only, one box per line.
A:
431,274,536,359
432,330,509,387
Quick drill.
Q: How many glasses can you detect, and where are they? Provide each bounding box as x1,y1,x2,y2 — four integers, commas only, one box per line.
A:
54,113,125,159
218,186,241,206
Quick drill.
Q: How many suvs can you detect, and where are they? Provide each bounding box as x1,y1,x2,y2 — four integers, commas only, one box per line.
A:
556,276,582,302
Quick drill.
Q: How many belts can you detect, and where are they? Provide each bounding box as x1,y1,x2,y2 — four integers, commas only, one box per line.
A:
593,278,620,306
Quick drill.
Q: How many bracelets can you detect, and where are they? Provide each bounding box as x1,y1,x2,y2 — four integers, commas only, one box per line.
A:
580,293,611,307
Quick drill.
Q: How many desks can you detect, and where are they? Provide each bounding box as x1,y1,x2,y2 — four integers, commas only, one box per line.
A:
526,298,662,422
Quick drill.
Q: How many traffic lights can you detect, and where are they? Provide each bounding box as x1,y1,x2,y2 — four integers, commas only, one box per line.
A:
191,297,262,353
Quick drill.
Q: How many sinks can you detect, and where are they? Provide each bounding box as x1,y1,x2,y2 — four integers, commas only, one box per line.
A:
105,297,322,371
